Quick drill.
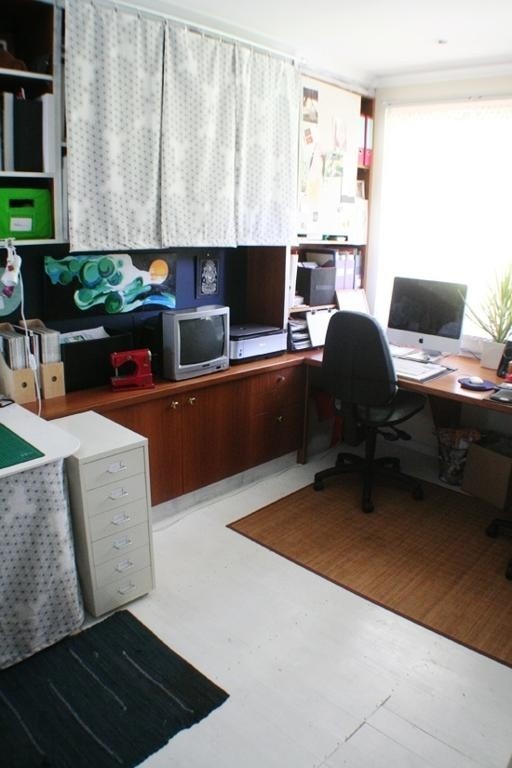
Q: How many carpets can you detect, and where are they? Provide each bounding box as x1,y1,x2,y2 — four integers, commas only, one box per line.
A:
0,610,229,768
226,467,512,668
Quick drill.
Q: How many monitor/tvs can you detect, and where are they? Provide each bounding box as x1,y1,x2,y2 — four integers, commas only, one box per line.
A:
142,304,230,382
387,276,469,365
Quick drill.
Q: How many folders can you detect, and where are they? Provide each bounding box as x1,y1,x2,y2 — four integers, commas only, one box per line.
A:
357,113,373,167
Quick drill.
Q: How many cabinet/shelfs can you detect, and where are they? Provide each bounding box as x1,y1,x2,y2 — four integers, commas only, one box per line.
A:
90,344,305,507
48,410,155,618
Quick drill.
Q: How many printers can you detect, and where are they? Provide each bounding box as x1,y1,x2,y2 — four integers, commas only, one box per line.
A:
232,319,288,363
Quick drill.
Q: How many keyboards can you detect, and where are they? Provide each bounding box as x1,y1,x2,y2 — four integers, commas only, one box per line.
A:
392,356,447,382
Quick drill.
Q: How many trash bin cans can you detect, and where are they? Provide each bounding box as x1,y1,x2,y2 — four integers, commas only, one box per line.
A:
437,427,482,486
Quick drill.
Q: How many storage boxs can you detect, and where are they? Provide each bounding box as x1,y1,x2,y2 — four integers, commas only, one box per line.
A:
295,266,335,307
462,433,512,510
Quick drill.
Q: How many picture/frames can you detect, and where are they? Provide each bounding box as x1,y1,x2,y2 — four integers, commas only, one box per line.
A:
195,256,222,299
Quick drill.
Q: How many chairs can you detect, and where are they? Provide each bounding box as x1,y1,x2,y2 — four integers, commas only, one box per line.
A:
313,312,426,513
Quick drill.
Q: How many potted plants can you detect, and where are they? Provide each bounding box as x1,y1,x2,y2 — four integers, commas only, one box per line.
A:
457,267,512,370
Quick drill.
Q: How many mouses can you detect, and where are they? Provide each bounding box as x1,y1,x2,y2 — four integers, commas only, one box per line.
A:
470,377,483,384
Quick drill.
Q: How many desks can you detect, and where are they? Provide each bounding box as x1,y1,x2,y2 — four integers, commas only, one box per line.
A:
0,390,84,670
297,349,512,465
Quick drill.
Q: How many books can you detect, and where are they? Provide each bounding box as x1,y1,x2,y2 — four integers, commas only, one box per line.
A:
356,179,366,200
302,246,363,290
289,319,311,351
0,323,112,371
